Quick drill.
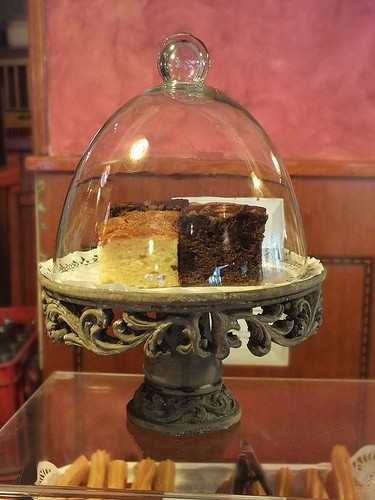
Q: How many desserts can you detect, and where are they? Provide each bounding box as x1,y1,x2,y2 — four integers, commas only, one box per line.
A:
178,202,267,287
94,209,180,290
108,198,185,215
